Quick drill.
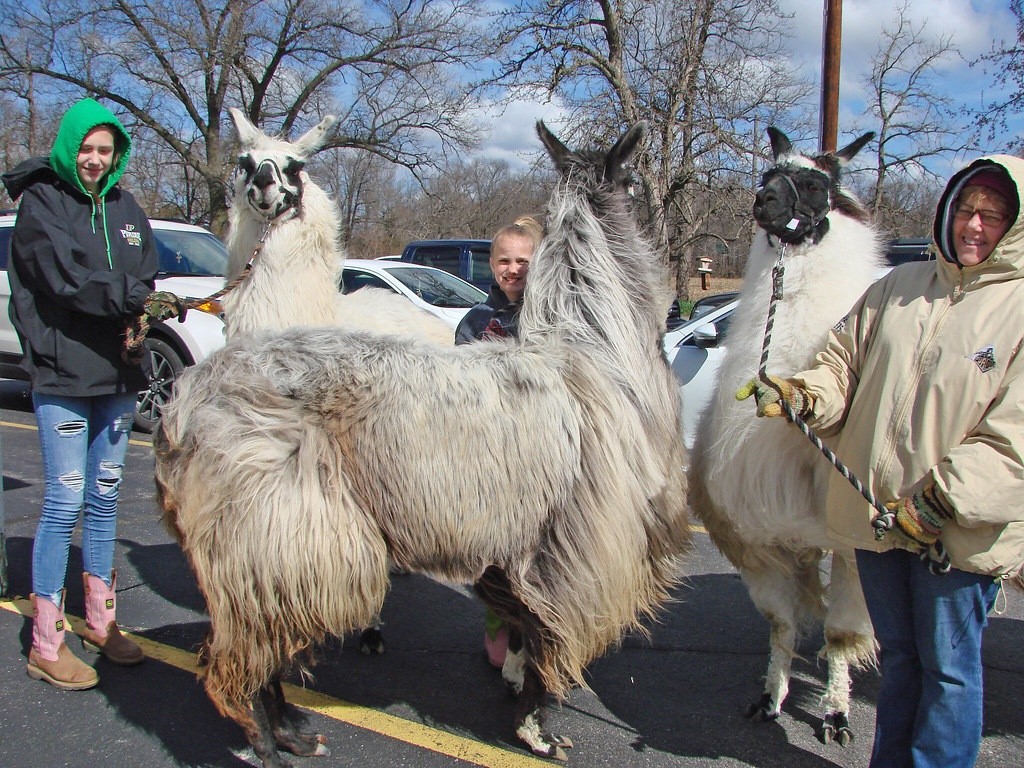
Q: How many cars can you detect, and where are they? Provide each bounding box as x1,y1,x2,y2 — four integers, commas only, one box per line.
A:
333,255,489,355
686,235,941,328
2,212,242,434
401,237,501,295
654,266,891,452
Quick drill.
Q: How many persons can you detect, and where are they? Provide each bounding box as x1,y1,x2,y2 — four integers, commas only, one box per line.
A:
736,153,1024,768
453,211,546,670
1,97,190,692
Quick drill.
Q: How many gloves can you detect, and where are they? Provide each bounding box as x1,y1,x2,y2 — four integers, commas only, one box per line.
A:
143,291,187,323
883,483,950,548
737,372,807,417
124,316,149,352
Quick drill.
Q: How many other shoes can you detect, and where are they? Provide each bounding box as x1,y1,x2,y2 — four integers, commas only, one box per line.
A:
484,618,505,666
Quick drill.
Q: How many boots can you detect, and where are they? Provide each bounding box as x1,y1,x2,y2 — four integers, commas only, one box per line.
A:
82,568,145,664
27,588,101,691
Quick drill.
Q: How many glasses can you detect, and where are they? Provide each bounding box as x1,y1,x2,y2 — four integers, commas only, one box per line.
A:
953,201,1014,228
